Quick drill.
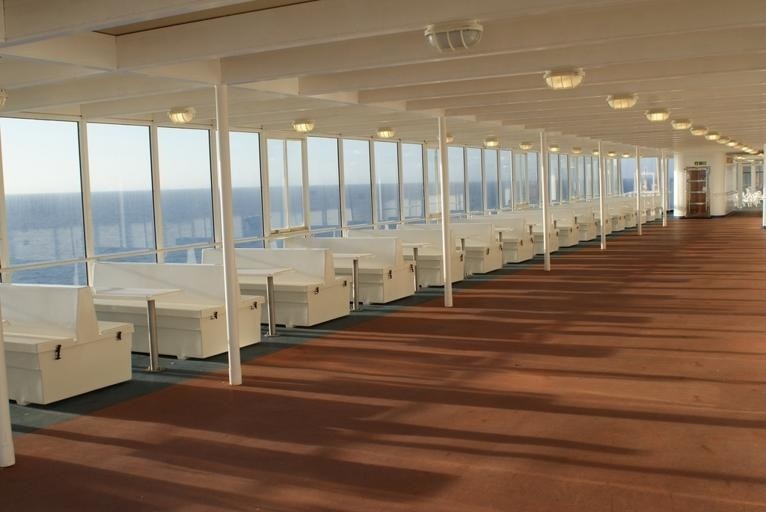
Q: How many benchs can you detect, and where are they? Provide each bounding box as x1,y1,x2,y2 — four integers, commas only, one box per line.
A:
0,189,665,406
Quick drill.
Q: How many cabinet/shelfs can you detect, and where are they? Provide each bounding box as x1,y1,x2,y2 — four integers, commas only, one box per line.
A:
684,166,713,218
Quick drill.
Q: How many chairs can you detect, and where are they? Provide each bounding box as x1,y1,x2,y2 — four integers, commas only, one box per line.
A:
737,184,762,213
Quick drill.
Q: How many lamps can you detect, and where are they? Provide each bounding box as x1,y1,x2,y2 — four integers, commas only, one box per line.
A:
518,141,532,150
621,151,629,157
541,67,586,91
549,145,561,154
166,104,401,142
690,125,761,155
424,21,486,52
482,135,500,148
672,117,691,130
608,150,617,156
592,149,601,156
436,133,454,143
643,108,672,122
570,146,581,154
604,91,638,109
